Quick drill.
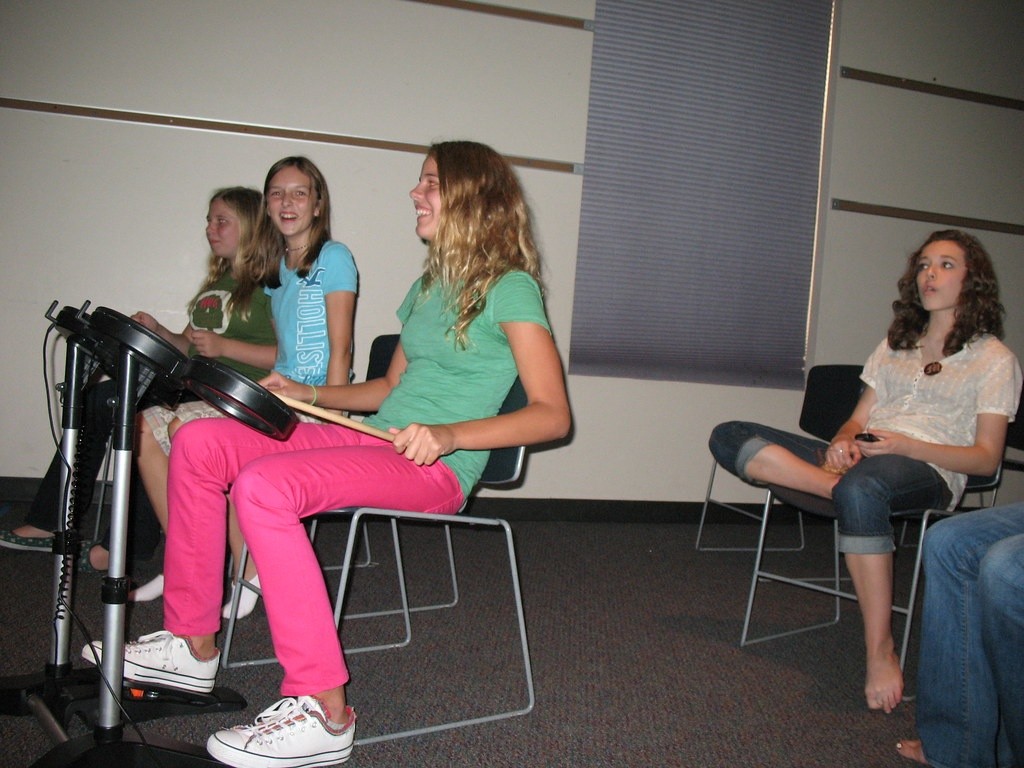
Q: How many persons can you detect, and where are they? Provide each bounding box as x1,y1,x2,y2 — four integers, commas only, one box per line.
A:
709,228,1024,716
81,142,574,768
126,157,358,619
895,500,1024,768
0,185,277,574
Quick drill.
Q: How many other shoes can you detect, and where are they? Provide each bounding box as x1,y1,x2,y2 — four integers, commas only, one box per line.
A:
74,541,109,578
0,529,53,553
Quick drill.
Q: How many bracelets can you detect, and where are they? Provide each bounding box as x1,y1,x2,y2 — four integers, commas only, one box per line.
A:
309,384,317,406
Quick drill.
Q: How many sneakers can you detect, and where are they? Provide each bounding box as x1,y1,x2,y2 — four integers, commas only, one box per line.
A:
82,629,221,693
207,695,356,768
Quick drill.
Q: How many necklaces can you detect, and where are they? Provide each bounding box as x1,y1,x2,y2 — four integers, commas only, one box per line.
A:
285,243,307,254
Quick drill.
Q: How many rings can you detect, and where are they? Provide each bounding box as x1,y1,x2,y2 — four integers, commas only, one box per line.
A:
839,449,842,453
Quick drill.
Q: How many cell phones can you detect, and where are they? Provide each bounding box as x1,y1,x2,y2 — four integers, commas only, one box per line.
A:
855,433,880,442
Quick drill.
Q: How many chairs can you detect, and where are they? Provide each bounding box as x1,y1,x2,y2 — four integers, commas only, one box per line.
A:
694,364,1024,704
221,335,536,748
